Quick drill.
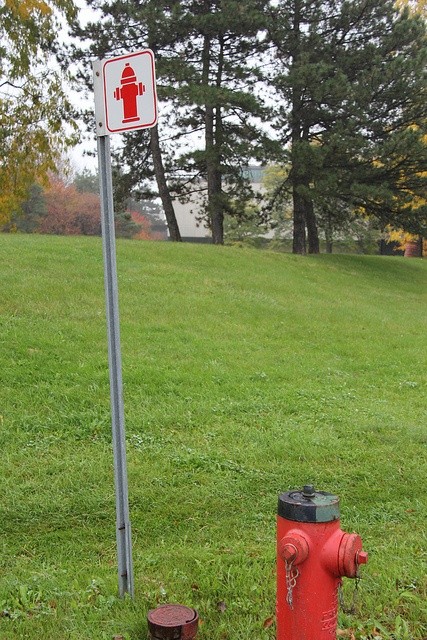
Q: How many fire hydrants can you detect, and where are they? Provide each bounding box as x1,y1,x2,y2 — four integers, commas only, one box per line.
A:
276,485,370,640
113,61,146,125
